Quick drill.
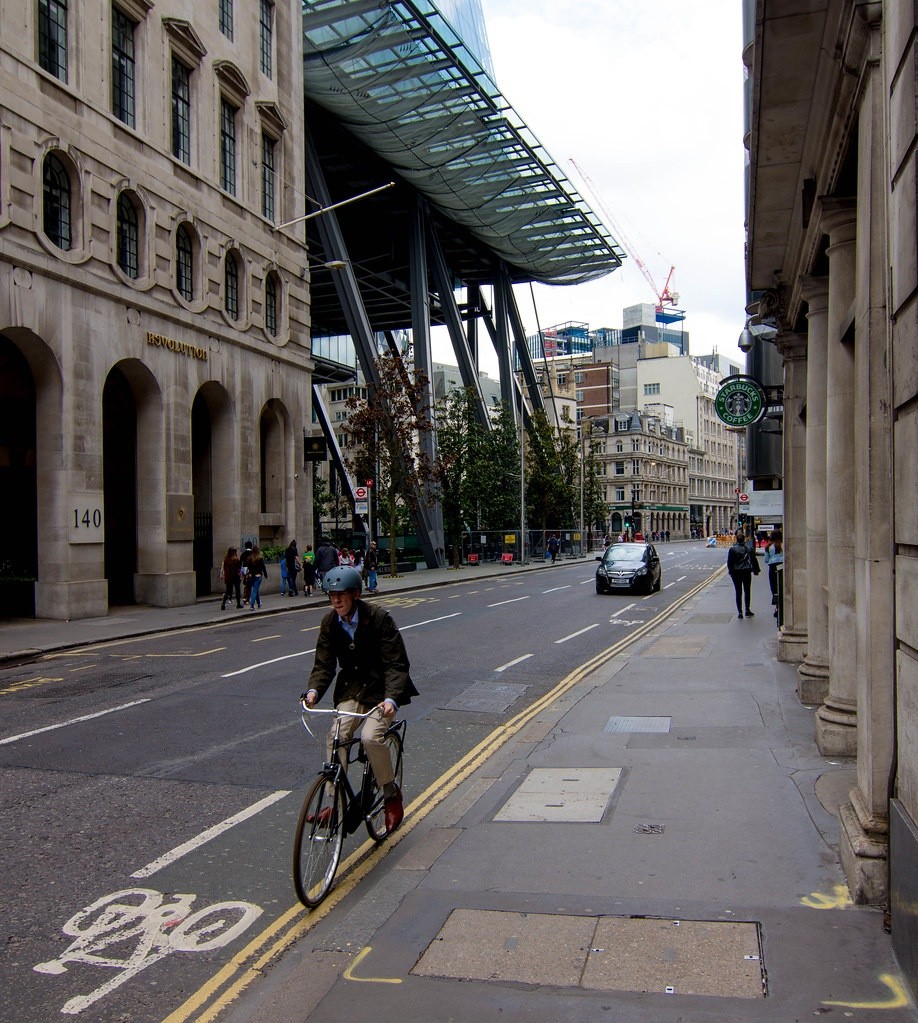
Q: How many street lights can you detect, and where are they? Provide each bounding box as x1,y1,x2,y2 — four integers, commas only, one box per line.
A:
580,414,601,556
521,381,546,567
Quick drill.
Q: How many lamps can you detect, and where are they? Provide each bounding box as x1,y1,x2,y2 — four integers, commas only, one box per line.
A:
300,261,346,277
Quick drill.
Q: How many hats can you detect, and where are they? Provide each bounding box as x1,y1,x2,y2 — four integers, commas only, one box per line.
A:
768,529,783,536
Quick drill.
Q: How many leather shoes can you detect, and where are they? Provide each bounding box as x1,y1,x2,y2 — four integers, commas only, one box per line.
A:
306,806,337,825
384,782,403,833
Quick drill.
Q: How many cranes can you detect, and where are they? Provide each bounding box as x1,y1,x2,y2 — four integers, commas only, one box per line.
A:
569,157,680,312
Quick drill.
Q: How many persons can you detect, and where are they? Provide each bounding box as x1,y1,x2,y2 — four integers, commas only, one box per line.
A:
728,532,761,618
692,527,701,539
645,530,671,543
765,529,783,618
602,533,624,551
281,540,379,597
756,530,762,549
221,541,268,611
546,533,560,564
301,566,420,834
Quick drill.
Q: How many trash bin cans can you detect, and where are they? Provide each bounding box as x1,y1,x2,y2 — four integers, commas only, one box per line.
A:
397,548,404,562
384,549,391,564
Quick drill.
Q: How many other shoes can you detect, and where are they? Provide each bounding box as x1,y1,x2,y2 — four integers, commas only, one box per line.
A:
250,608,257,611
305,592,307,596
243,598,249,604
745,611,754,617
258,603,262,608
369,589,378,592
288,593,294,597
221,605,225,610
738,615,743,619
237,605,244,609
281,592,286,596
295,593,299,596
310,594,314,597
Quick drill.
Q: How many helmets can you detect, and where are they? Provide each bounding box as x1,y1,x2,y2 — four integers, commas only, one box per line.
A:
321,566,362,593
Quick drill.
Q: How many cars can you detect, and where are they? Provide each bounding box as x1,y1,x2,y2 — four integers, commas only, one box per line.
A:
595,542,661,596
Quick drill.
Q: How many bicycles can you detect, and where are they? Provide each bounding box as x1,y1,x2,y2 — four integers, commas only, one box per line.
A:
292,690,410,908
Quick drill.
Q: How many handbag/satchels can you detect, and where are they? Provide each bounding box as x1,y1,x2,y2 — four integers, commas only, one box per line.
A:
240,566,249,575
740,553,754,573
220,561,225,579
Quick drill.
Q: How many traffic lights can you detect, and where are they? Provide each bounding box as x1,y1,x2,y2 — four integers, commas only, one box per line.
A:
624,515,634,527
